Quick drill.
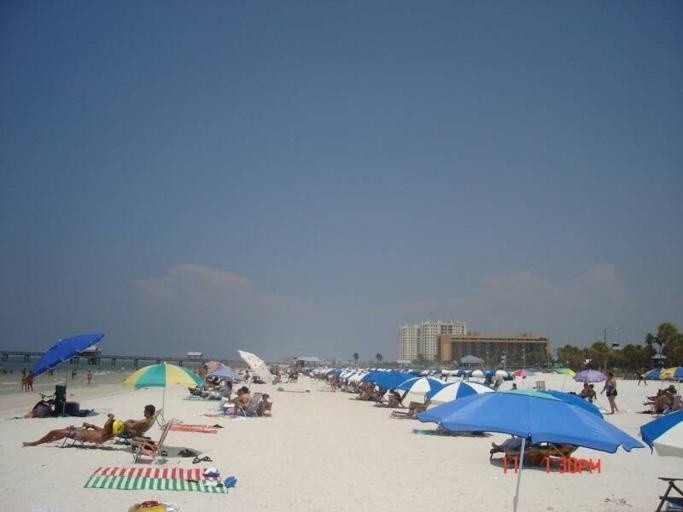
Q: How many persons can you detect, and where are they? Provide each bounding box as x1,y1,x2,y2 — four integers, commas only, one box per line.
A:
651,385,681,415
23,414,124,446
192,366,269,416
124,405,155,436
20,369,28,391
86,369,93,384
26,371,33,392
272,363,299,383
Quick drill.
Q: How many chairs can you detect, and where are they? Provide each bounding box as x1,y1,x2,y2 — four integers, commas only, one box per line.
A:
60,408,174,466
489,442,578,467
236,393,262,417
39,385,66,417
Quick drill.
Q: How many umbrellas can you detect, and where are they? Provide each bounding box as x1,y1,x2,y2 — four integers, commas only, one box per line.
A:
31,332,105,416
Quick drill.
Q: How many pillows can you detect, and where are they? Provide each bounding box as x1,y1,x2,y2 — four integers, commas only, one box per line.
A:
201,467,237,488
